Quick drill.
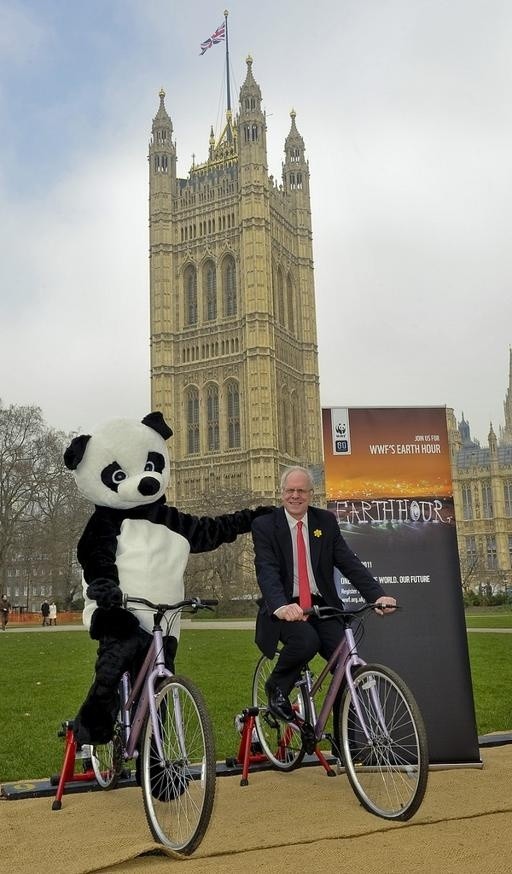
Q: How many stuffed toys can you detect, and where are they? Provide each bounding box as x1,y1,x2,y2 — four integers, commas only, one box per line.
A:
63,412,276,802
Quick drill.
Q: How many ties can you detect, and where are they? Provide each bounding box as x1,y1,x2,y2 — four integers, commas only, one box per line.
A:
296,521,312,618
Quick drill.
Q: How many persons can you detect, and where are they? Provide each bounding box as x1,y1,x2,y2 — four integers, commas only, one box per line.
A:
251,466,397,768
41,600,50,626
0,594,12,631
49,602,57,625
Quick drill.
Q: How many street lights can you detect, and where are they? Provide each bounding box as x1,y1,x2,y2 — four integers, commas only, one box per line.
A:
501,576,509,594
19,554,30,610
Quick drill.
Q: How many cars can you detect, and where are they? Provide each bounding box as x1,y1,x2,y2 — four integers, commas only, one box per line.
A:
476,585,502,601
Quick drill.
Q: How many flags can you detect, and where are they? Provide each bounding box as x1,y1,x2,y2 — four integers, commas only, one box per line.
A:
198,22,225,55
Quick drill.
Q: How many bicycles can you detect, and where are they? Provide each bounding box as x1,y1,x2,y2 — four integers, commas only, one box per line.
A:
249,602,429,822
84,593,227,855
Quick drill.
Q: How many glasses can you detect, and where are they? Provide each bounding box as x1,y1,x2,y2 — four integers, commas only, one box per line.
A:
285,487,313,494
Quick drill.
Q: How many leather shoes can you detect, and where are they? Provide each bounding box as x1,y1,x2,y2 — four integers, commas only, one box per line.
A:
265,679,294,721
331,745,362,762
